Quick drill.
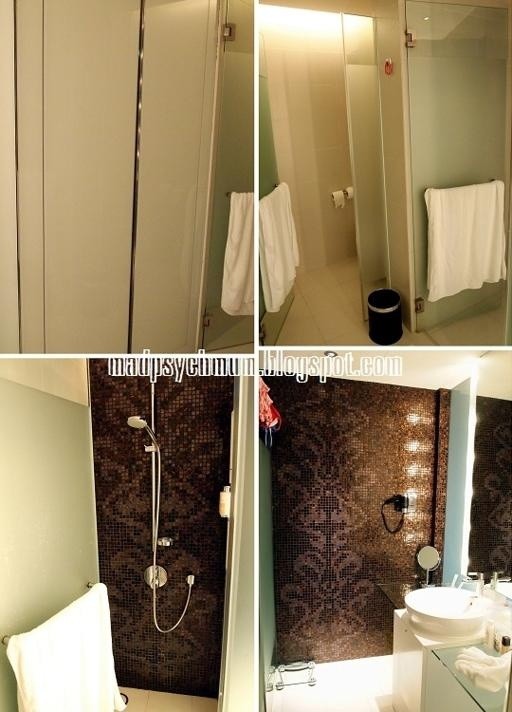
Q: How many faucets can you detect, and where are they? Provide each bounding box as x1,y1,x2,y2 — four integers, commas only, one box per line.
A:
490,569,511,591
465,570,486,609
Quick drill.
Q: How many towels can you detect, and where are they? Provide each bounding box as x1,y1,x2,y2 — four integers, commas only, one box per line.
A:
457,646,512,693
424,180,506,302
219,193,252,317
4,583,132,712
259,182,300,312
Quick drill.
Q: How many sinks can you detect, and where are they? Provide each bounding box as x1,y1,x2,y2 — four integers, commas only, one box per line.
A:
485,581,512,600
405,585,487,639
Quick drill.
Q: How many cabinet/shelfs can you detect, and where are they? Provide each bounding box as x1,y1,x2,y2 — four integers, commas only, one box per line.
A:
391,611,507,711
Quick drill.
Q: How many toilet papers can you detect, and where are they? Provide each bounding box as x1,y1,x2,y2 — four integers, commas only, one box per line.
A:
330,190,345,209
347,185,354,199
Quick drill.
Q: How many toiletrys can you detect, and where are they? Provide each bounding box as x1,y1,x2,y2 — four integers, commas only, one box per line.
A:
484,619,510,655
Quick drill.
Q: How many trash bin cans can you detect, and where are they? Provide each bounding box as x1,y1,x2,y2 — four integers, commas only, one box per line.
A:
368,289,403,344
